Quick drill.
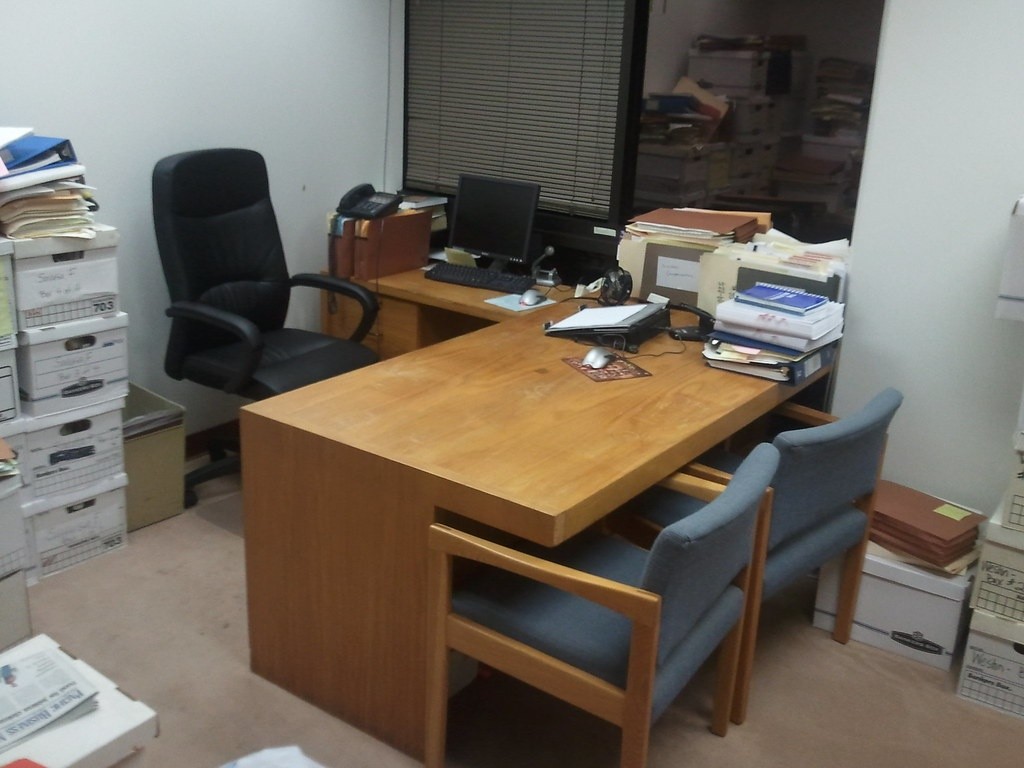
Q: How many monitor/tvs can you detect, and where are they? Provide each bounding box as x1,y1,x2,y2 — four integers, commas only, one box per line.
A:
449,173,540,274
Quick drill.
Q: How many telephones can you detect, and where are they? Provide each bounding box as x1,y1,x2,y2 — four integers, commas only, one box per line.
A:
335,182,404,221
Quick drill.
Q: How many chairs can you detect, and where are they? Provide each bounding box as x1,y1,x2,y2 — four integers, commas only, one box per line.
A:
151,149,381,508
424,387,904,768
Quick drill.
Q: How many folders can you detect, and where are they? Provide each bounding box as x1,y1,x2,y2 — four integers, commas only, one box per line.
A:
707,344,835,388
0,133,80,181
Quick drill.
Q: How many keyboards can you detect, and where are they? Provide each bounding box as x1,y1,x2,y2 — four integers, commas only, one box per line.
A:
424,261,536,295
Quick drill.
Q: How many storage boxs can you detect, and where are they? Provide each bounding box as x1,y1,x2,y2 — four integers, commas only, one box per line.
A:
956,433,1024,722
996,194,1024,322
0,224,128,582
632,48,873,213
0,634,159,768
813,551,977,672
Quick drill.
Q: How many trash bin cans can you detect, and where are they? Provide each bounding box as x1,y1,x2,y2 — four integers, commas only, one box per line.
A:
121,379,188,532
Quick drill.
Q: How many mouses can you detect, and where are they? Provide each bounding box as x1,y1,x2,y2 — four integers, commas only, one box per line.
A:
518,289,547,306
581,346,613,370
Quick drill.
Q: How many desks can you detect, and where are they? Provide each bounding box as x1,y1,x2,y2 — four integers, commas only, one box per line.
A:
240,293,835,764
320,264,576,361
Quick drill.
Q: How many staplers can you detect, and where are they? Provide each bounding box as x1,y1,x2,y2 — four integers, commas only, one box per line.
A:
669,300,716,342
